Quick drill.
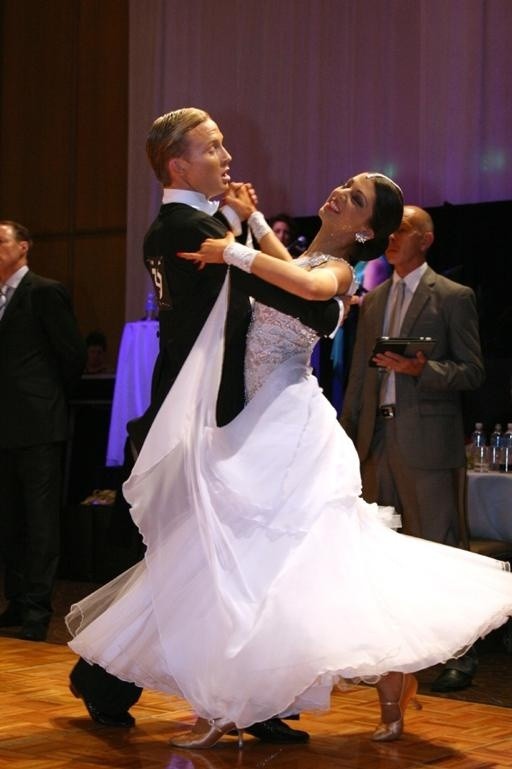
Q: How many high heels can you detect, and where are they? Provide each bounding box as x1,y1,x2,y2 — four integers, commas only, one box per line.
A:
372,673,422,739
170,712,245,752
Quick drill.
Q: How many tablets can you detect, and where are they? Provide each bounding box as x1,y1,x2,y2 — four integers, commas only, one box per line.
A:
367,336,438,367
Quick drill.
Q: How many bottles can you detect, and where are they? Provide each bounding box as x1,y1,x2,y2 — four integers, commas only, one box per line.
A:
145,293,157,320
468,422,511,473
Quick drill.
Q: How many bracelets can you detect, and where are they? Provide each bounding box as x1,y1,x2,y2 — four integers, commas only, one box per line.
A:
248,211,272,242
223,243,262,273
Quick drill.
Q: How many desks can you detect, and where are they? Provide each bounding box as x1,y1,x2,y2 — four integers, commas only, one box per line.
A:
466,470,512,544
105,320,160,467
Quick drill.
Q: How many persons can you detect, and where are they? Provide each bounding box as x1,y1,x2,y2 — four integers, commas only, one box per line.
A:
63,173,511,750
271,214,293,246
84,331,115,374
69,104,359,746
339,206,492,691
1,219,88,640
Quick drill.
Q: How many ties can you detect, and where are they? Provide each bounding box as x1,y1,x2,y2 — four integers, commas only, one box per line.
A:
379,282,408,408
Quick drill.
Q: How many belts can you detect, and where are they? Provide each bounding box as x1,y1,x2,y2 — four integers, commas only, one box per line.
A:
372,405,396,419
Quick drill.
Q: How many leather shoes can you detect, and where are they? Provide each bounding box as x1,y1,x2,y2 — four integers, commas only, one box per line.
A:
438,665,474,692
68,679,136,728
1,607,25,628
20,625,45,641
224,709,311,746
